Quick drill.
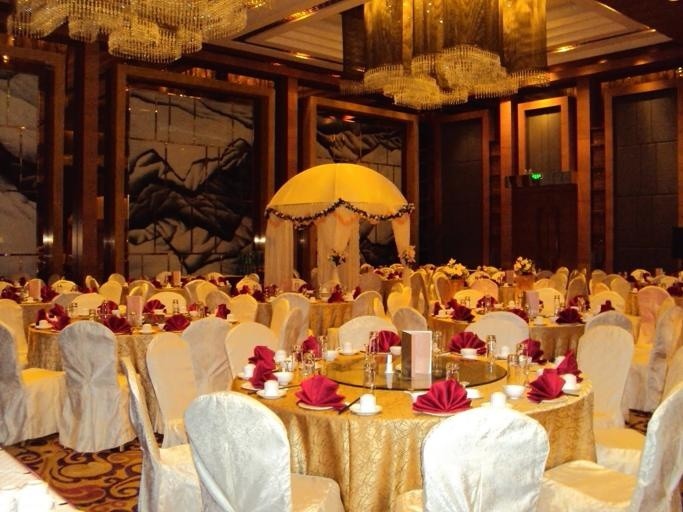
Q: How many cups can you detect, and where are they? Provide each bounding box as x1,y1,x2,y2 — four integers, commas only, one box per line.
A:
30,302,242,334
434,294,602,324
233,330,583,417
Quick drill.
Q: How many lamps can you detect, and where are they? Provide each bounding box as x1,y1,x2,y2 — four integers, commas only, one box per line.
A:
6,0,266,66
358,0,550,112
